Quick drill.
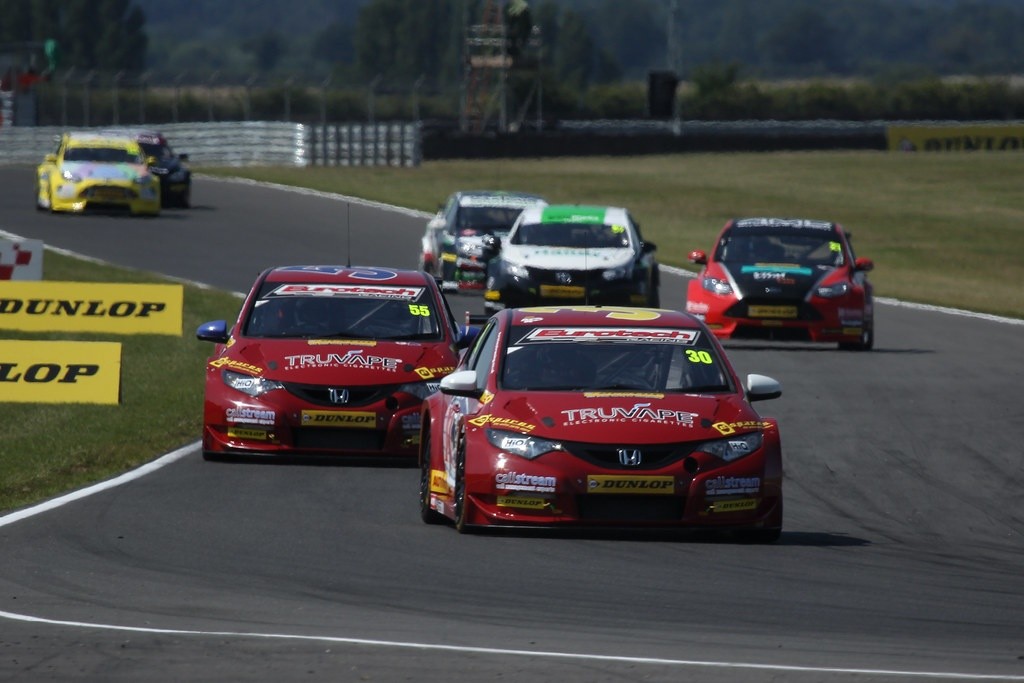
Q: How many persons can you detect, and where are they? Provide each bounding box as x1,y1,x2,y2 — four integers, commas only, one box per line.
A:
296,299,334,331
527,345,582,386
725,234,753,260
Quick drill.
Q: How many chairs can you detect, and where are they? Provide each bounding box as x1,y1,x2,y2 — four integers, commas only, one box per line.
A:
294,297,331,334
509,350,553,388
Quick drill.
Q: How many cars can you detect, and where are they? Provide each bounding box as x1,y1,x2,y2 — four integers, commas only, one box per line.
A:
418,303,784,540
475,204,657,310
195,266,482,464
110,132,193,211
37,131,161,218
686,217,875,350
422,191,547,290
